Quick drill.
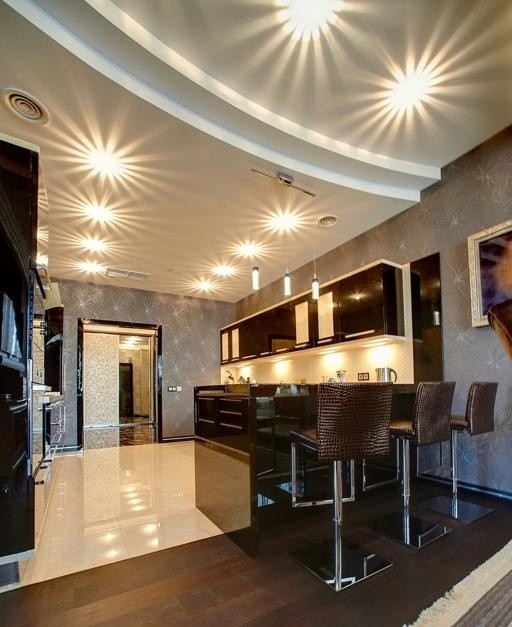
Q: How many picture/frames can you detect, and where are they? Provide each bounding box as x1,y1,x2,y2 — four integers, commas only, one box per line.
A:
467,220,512,328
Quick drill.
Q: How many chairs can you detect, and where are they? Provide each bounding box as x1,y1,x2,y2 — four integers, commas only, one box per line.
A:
369,382,456,552
420,382,496,526
290,383,393,591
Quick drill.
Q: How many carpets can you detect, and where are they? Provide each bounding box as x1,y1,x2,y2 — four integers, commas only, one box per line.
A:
400,538,512,627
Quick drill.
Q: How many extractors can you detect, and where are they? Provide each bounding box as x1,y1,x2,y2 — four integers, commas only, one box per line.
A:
1,289,26,373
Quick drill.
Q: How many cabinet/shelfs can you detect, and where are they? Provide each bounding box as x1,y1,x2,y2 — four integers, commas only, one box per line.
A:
32,149,66,558
220,263,405,366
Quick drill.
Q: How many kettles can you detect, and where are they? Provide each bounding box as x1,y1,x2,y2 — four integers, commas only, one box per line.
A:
376,367,398,383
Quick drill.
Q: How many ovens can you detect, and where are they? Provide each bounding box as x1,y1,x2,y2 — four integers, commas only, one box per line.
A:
0,398,28,479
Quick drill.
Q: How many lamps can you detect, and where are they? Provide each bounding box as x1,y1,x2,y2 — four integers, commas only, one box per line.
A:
250,167,320,301
